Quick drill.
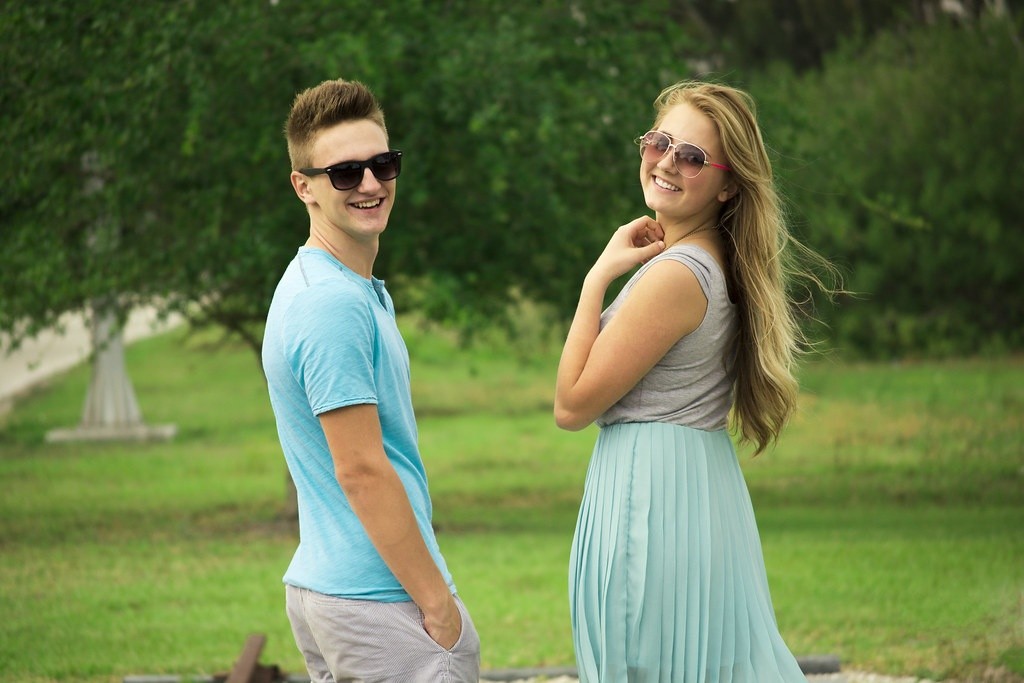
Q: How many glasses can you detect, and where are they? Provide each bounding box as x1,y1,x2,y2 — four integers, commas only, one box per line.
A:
299,149,403,190
634,131,733,179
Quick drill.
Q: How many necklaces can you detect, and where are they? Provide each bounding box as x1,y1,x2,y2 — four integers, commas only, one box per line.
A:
659,227,711,253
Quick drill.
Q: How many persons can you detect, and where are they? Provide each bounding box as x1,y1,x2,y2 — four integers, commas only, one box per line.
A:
554,80,829,683
259,77,484,683
